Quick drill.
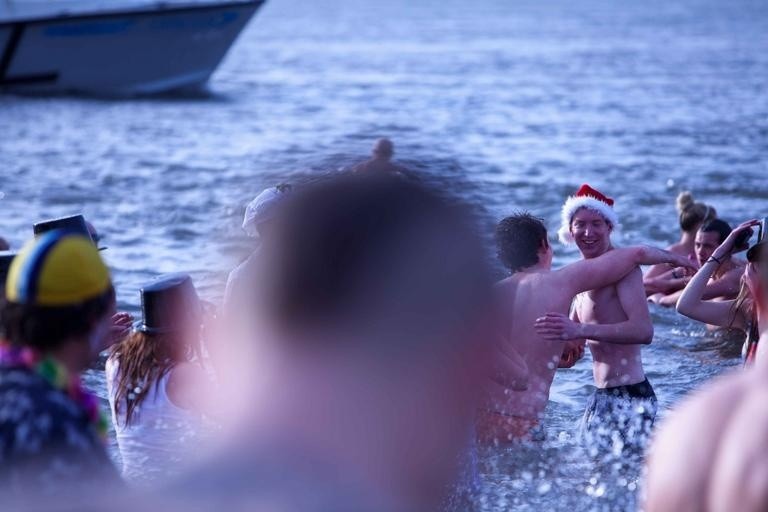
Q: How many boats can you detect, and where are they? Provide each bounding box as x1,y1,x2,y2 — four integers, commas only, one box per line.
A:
0,0,265,96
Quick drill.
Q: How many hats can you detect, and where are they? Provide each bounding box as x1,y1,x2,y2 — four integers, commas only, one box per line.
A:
558,185,618,246
131,276,213,336
4,213,111,307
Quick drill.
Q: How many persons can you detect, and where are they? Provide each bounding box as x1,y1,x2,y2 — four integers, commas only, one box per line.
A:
639,187,768,512
469,318,530,439
480,209,700,465
533,181,661,465
1,138,504,512
